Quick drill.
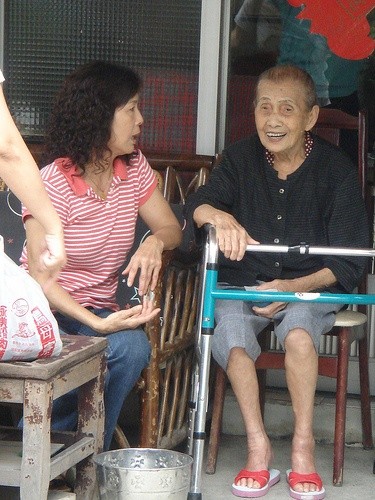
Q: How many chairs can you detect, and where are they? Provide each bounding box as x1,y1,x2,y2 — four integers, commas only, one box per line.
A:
205,108,373,486
0,145,217,449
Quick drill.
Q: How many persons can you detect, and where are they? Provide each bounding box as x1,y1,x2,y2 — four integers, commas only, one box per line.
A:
20,62,182,452
230,0,375,165
0,70,67,274
187,65,371,500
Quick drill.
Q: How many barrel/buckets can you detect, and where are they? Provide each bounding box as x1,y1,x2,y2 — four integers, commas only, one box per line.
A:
92,449,193,500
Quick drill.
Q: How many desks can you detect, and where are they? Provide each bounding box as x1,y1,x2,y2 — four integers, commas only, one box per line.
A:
0,335,108,500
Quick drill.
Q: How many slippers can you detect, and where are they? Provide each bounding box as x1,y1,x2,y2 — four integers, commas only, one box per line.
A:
287,469,326,500
231,469,281,497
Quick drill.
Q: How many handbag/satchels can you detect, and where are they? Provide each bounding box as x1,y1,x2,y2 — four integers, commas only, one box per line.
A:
0,235,63,361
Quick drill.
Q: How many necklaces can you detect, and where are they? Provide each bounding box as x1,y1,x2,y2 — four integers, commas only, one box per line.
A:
265,131,313,169
86,167,111,198
